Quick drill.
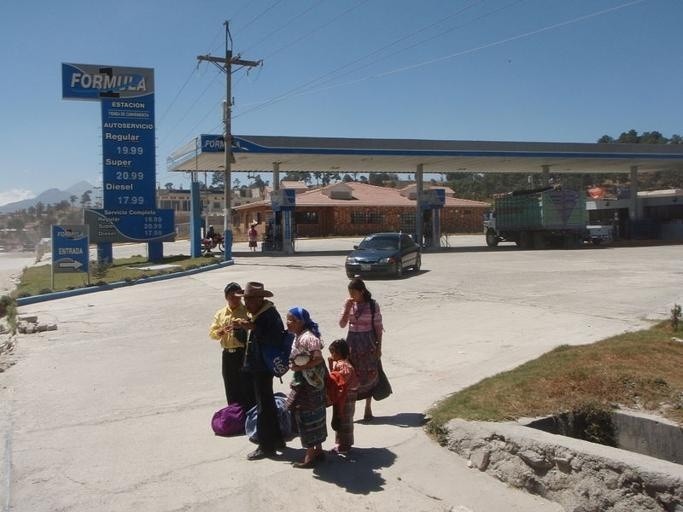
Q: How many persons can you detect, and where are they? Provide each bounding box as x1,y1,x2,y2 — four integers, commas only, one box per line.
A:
285,307,330,470
204,224,216,253
337,277,385,423
208,281,257,415
234,280,287,462
247,223,258,252
326,338,362,454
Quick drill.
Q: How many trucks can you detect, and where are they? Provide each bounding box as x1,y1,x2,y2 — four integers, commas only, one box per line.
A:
481,185,589,250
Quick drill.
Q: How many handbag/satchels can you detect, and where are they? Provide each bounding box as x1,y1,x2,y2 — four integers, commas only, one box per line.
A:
257,311,294,385
211,403,244,436
372,362,392,401
244,392,298,445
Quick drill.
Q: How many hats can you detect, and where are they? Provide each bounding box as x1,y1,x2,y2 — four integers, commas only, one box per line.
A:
234,281,273,298
224,281,241,298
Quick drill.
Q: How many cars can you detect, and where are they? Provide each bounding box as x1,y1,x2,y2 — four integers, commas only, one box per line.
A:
344,231,422,279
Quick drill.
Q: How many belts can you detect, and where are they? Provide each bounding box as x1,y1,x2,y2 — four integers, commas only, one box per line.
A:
224,348,242,353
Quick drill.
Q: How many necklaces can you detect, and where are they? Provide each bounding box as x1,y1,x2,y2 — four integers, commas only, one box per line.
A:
352,300,366,320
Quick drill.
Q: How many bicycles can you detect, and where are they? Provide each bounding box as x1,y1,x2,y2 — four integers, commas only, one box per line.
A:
200,232,224,253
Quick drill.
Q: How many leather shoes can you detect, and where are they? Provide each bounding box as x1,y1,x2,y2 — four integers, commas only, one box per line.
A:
247,448,275,461
294,459,313,468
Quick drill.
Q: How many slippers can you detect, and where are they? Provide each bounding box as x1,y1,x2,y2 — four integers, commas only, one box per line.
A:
328,447,349,454
